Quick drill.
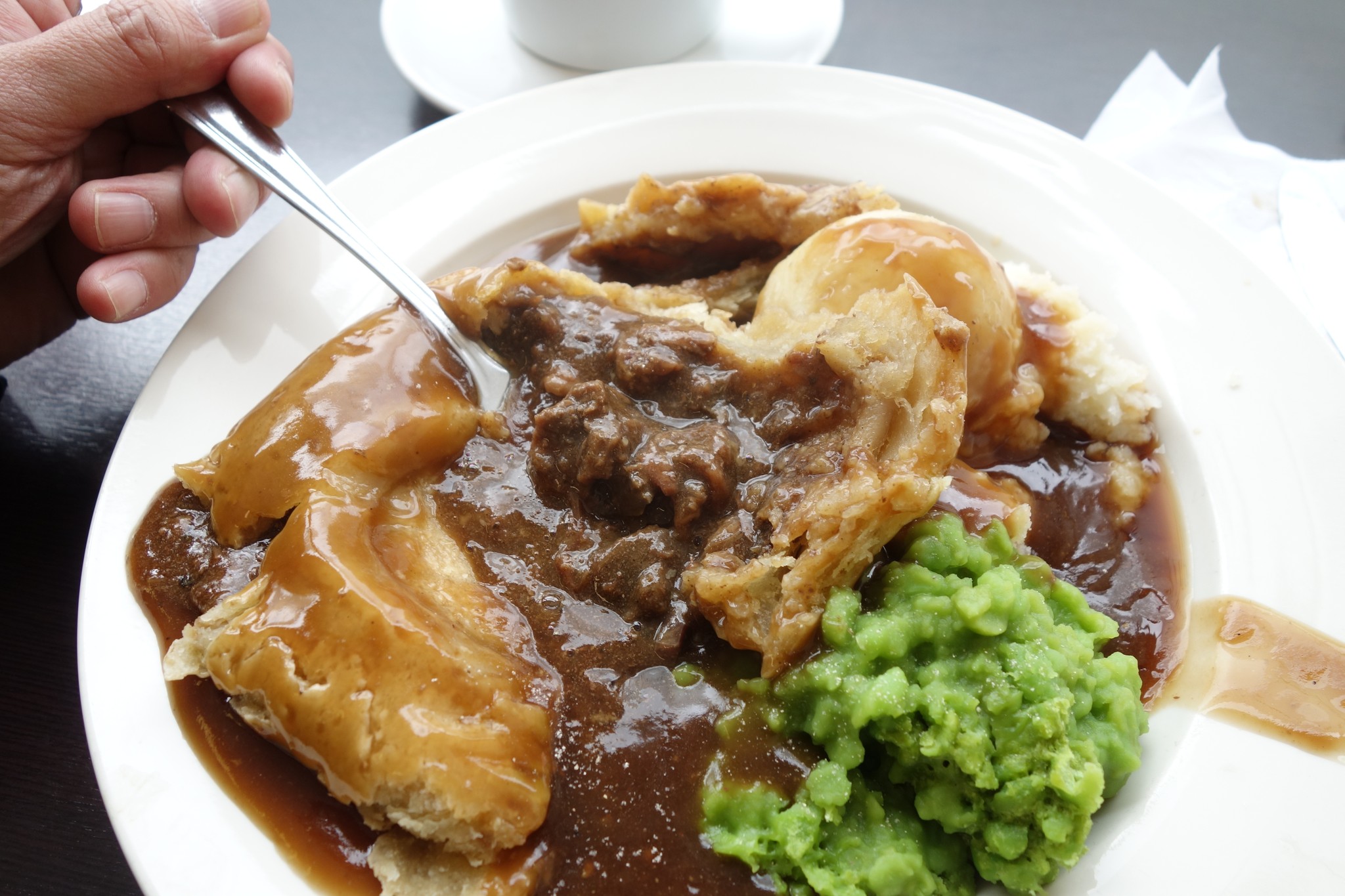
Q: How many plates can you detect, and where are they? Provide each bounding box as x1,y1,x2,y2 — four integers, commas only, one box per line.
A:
76,62,1345,896
378,0,844,115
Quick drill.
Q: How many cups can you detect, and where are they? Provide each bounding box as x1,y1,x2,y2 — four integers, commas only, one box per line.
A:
504,0,718,72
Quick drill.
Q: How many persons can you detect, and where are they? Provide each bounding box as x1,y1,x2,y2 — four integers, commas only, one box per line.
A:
0,0,294,368
